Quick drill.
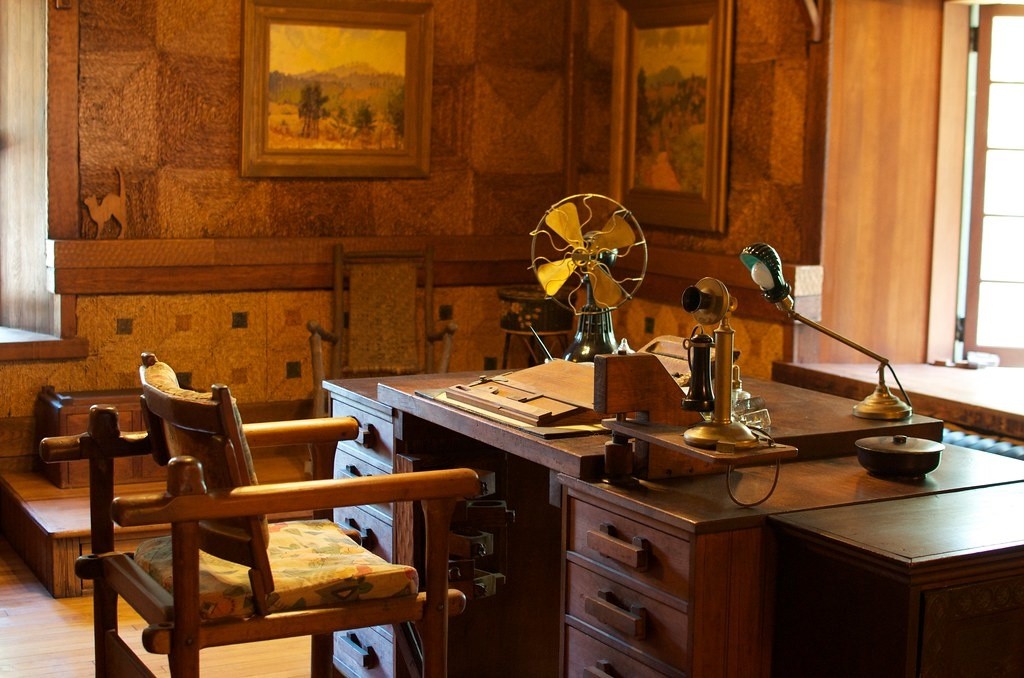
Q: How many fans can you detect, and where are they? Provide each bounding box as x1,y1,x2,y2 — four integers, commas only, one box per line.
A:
529,193,646,360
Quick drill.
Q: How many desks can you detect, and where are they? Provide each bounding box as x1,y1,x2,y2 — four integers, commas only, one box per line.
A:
379,360,944,478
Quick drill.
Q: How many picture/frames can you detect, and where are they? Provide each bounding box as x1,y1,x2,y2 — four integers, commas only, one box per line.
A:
610,0,732,236
238,0,432,180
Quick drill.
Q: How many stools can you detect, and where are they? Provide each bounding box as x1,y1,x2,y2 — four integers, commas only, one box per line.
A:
501,331,576,367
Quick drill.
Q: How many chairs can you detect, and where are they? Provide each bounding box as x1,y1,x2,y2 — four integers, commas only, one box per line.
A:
312,243,454,416
42,355,481,678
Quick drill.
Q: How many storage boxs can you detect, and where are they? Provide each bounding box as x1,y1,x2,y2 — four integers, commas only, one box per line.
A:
501,286,576,328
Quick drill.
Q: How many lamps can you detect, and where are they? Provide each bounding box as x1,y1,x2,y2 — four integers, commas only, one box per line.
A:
739,245,913,422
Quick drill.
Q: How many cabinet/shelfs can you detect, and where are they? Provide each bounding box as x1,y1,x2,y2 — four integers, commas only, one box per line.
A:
0,460,310,596
556,445,1023,677
40,391,179,488
767,481,1024,677
311,379,567,678
